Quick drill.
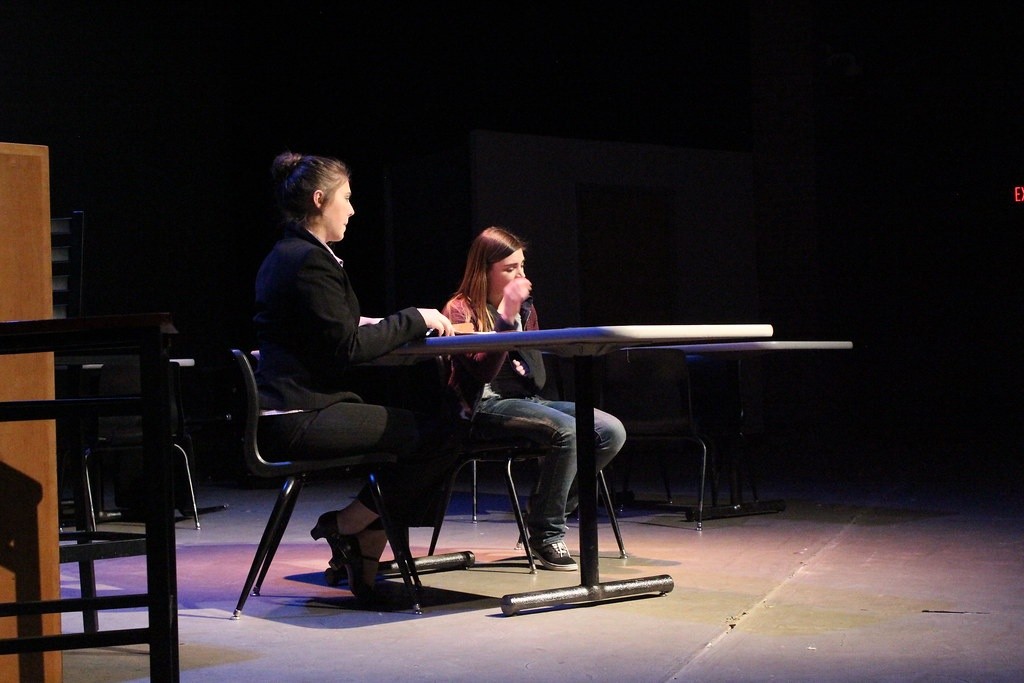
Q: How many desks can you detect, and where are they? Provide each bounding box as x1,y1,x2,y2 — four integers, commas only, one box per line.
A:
324,323,774,613
621,341,853,521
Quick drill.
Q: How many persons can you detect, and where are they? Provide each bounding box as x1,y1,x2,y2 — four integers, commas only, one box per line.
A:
442,227,626,571
255,153,456,606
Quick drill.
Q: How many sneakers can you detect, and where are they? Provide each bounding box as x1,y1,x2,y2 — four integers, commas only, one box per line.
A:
527,537,578,571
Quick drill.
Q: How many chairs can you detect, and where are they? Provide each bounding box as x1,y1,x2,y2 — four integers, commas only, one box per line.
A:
229,350,424,620
82,354,201,530
428,346,763,574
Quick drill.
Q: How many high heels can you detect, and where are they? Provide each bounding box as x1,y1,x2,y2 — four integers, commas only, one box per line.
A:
310,510,349,582
328,535,382,606
